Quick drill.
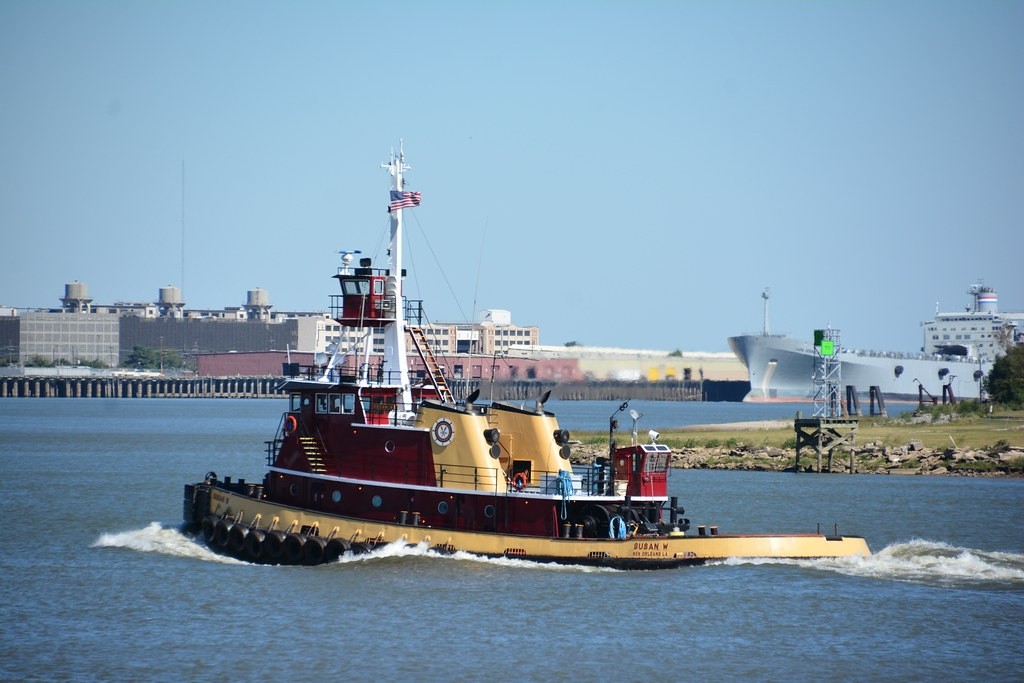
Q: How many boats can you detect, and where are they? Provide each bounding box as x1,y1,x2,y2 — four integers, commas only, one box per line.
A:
726,285,996,405
181,136,874,567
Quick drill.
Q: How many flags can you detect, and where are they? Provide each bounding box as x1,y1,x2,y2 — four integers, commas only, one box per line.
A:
390,191,421,211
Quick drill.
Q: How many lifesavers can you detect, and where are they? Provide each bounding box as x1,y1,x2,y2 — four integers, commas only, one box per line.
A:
512,472,527,489
284,415,298,434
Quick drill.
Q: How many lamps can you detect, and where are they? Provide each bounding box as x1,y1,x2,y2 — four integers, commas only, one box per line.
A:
648,429,660,441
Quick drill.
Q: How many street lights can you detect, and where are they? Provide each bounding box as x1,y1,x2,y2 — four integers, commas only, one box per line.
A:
159,336,165,374
